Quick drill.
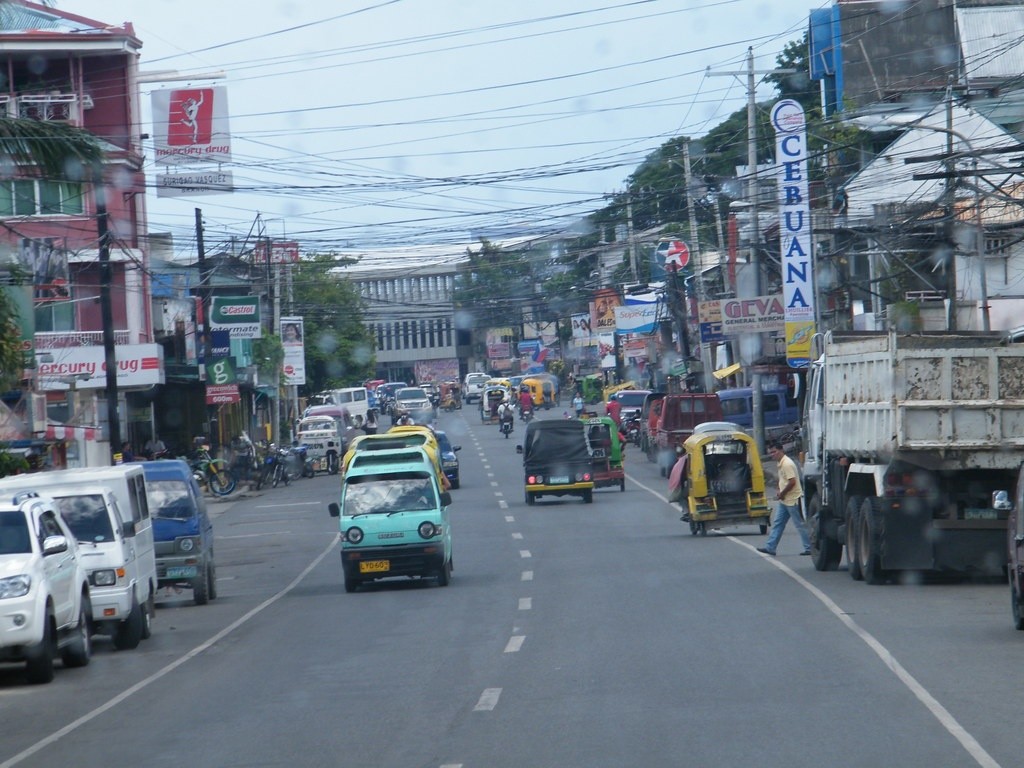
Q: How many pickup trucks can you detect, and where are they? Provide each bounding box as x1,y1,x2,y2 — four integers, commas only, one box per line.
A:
656,393,726,475
708,386,806,445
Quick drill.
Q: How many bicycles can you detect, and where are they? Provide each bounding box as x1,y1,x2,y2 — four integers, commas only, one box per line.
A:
217,433,317,490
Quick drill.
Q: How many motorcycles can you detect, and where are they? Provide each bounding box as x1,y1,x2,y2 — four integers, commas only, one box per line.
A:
623,410,640,445
516,394,537,423
498,417,514,440
513,419,596,504
576,419,628,492
156,440,234,497
668,420,773,535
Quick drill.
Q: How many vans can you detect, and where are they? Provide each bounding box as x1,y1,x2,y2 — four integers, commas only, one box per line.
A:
327,447,454,590
301,405,357,452
645,399,662,461
640,393,666,452
304,388,368,430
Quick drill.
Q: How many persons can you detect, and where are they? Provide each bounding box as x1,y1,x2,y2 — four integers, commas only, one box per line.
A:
145,432,166,462
360,342,663,438
226,433,256,484
121,441,145,463
281,324,301,345
574,315,595,338
757,442,814,556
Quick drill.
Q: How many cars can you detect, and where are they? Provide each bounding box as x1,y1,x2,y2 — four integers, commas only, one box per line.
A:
991,458,1024,632
364,378,462,426
428,430,461,488
609,389,652,432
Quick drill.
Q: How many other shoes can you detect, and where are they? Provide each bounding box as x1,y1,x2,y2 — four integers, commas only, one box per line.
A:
800,550,811,556
754,548,776,557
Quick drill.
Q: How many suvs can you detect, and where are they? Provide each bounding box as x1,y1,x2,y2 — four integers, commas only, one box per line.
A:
464,369,557,420
0,490,95,683
125,463,215,605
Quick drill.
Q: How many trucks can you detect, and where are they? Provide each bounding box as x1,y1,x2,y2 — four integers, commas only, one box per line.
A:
794,329,1024,587
334,427,451,501
2,463,157,647
292,415,343,476
